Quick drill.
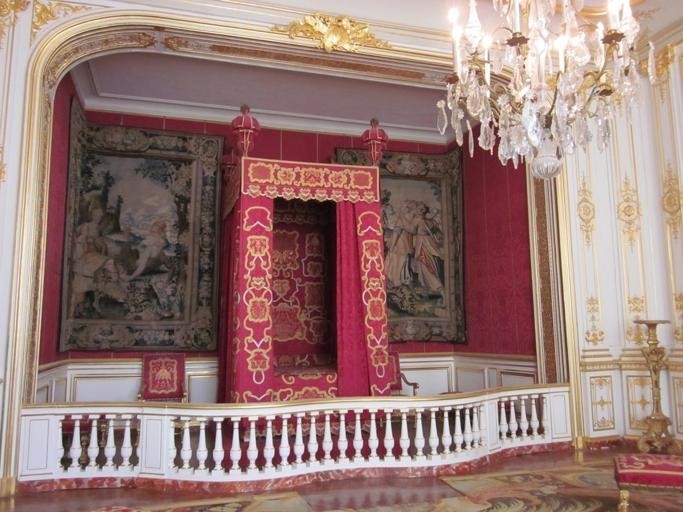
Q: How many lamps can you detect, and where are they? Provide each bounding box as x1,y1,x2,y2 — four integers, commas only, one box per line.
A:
433,3,650,179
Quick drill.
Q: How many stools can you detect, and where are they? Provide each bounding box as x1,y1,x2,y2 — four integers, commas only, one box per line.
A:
612,453,683,511
60,413,107,464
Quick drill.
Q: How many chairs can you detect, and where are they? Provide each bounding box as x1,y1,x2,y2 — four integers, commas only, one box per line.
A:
135,349,189,445
386,352,426,430
